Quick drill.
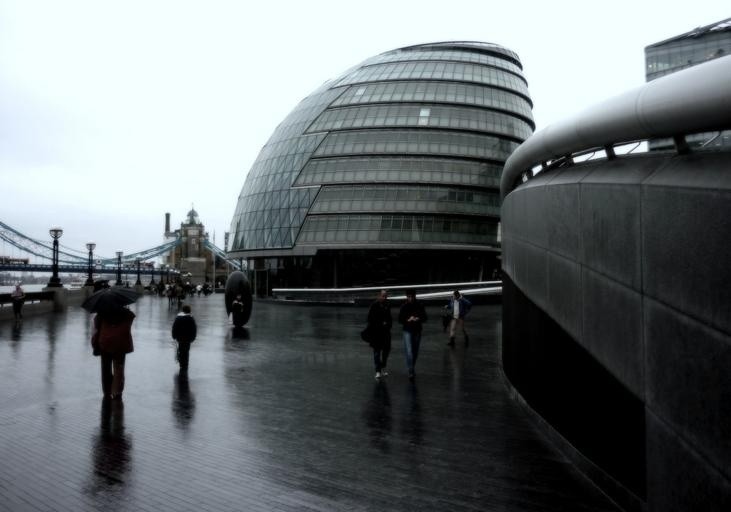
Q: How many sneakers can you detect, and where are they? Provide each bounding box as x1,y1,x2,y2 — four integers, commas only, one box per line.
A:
407,367,416,379
375,367,389,378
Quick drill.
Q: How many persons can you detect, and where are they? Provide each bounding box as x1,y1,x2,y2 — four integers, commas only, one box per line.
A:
398,379,425,451
92,395,128,486
13,321,22,342
398,289,428,378
366,379,392,453
366,290,392,378
11,283,26,322
232,294,244,329
172,306,196,373
95,306,136,402
172,372,196,430
92,281,222,306
445,291,472,346
447,345,469,399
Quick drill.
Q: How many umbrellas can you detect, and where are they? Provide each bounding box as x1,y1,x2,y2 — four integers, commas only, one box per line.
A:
82,287,140,313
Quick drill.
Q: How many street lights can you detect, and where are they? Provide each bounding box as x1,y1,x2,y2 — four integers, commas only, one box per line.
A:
150,260,180,282
86,241,96,286
116,250,123,285
47,226,63,287
135,255,142,285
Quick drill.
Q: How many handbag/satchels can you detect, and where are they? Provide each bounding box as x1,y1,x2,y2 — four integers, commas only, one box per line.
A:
91,330,101,356
361,325,385,350
